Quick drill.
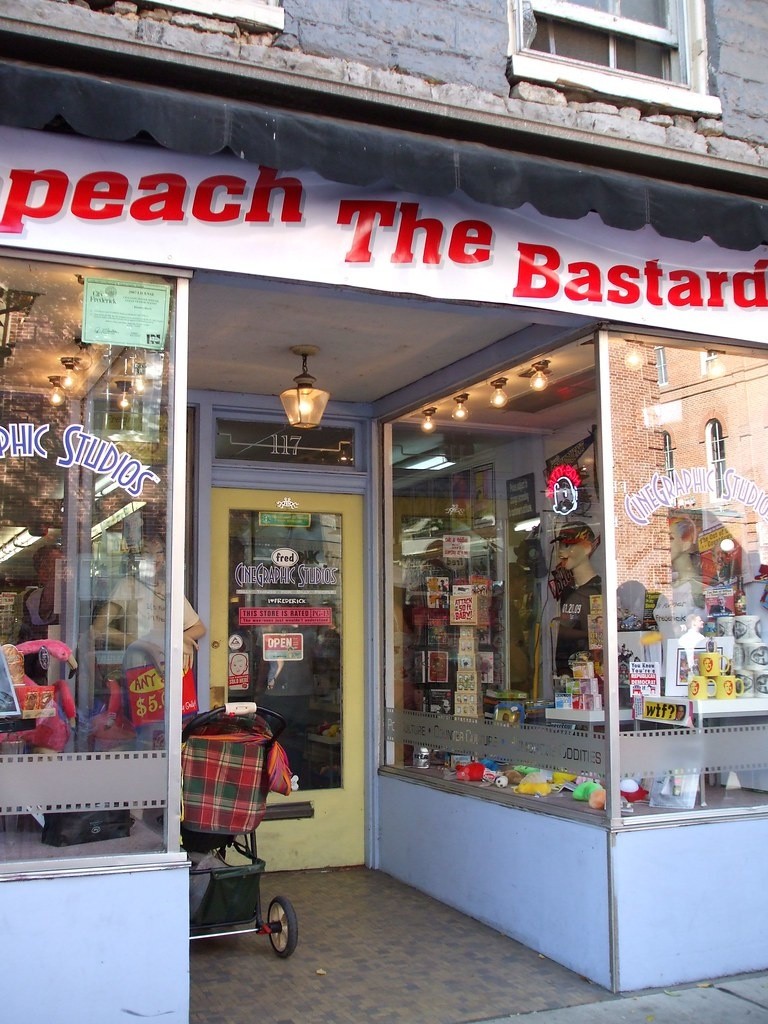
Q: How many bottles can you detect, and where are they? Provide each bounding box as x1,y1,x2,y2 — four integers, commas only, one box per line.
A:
734,577,746,615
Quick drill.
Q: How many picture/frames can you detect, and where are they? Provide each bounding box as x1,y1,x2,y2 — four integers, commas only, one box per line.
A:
393,486,414,544
471,459,497,529
431,473,451,536
411,477,431,539
664,635,734,696
449,464,473,533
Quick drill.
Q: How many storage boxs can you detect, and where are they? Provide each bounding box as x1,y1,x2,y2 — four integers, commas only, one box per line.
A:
484,594,604,710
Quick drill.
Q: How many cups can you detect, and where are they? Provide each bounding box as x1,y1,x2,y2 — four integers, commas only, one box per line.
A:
688,616,768,700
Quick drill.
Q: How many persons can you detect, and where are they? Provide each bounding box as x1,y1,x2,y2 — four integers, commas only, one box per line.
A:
709,595,732,616
677,613,709,649
262,589,330,790
653,512,718,676
15,545,66,686
549,521,602,676
90,532,206,677
708,543,741,590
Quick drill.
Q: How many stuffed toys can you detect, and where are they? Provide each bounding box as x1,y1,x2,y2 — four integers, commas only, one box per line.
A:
454,759,649,810
0,638,77,750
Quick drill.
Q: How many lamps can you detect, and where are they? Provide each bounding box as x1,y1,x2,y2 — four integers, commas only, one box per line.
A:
0,527,49,563
451,393,470,421
490,377,509,408
529,361,550,391
393,445,464,471
421,407,438,433
114,380,133,411
48,375,66,406
60,357,81,391
279,345,331,429
73,337,93,370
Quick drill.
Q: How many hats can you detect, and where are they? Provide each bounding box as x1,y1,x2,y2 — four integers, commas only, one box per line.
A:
550,521,595,547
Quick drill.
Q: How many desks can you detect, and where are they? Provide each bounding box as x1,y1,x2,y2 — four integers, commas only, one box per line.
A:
545,708,660,737
633,695,768,807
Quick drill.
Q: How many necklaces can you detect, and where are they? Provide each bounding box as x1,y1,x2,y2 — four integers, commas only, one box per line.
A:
132,575,165,601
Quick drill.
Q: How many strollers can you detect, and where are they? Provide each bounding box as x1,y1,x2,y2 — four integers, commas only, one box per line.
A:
183,703,300,959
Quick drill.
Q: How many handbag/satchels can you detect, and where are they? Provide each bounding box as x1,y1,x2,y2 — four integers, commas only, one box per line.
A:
41,809,135,847
